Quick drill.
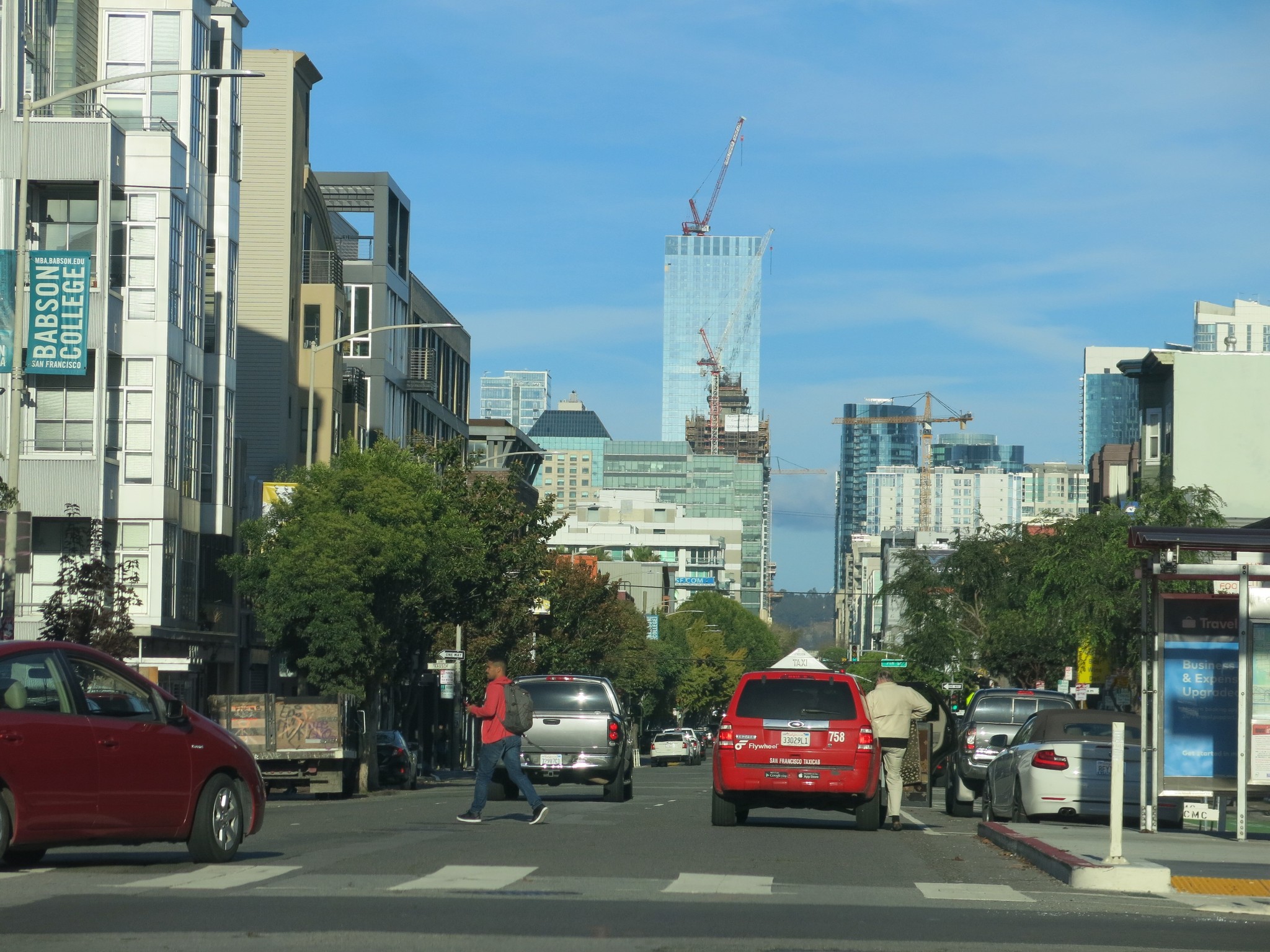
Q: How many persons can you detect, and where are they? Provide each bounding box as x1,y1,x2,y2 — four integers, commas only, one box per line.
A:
456,655,550,825
865,671,933,831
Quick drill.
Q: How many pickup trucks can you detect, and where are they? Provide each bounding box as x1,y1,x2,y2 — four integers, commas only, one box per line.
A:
662,728,702,764
499,674,636,802
945,687,1080,819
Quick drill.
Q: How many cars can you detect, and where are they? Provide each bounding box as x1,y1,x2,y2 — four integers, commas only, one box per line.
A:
982,708,1187,830
0,640,267,874
372,730,418,788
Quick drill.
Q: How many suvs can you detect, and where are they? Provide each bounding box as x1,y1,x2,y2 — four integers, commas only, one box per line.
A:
693,731,706,761
648,732,696,766
710,669,884,831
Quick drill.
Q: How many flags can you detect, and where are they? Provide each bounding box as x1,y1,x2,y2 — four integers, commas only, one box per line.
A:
260,480,302,558
646,615,659,640
23,250,92,376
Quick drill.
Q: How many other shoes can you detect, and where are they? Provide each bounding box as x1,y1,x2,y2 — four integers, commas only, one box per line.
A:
892,818,902,830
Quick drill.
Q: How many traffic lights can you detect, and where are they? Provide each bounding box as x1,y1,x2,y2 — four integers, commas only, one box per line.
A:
849,645,860,662
951,693,959,712
838,667,846,675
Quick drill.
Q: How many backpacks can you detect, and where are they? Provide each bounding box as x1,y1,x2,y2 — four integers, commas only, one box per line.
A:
496,682,533,734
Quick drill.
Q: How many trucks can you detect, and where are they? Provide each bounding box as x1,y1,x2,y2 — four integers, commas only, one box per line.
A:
199,689,361,799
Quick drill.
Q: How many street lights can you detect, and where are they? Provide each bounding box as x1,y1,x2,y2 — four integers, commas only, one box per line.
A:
452,448,568,683
0,64,262,641
305,318,462,476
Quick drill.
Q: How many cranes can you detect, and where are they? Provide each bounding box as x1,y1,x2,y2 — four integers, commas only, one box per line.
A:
832,390,972,528
765,454,827,476
682,114,746,237
697,227,774,455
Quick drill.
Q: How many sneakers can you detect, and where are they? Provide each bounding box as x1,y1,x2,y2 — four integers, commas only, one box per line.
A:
456,809,481,823
529,804,549,825
